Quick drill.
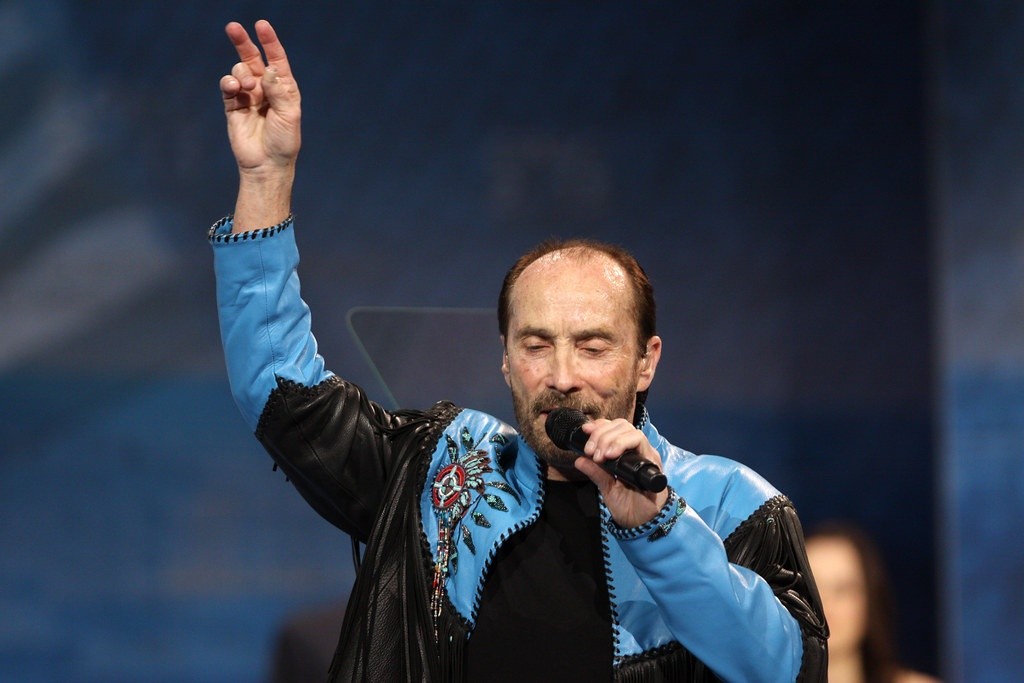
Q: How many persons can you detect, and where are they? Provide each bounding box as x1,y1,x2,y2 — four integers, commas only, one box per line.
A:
803,525,937,682
206,19,831,683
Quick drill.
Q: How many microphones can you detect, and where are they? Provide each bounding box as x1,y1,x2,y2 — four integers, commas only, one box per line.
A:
545,406,668,494
